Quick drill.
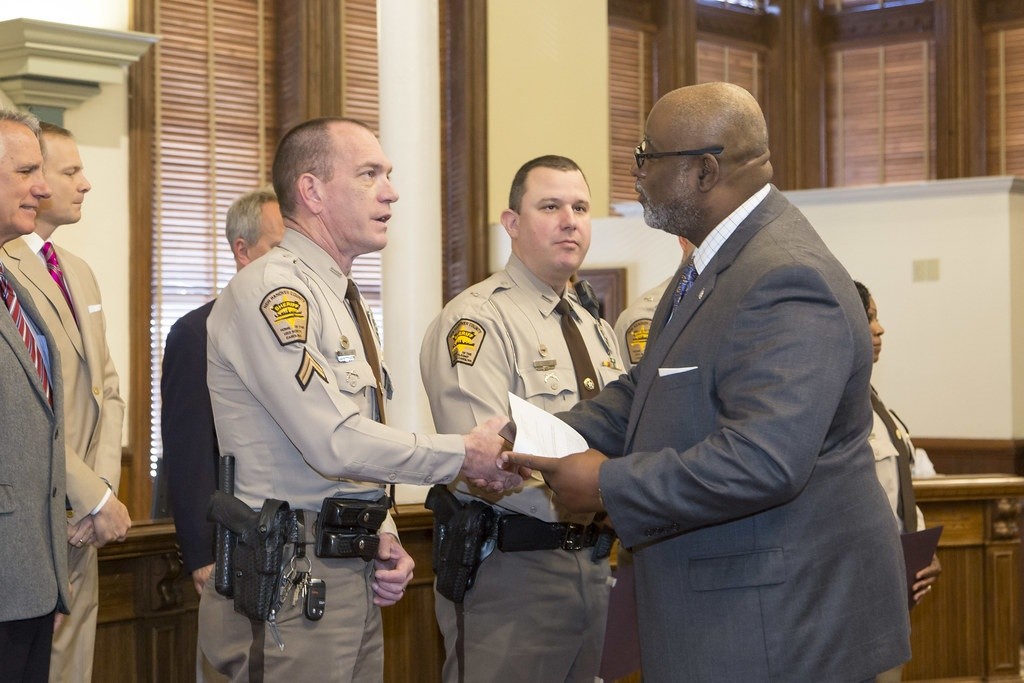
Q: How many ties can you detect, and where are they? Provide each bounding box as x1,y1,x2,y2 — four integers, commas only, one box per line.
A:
666,255,697,325
0,261,56,412
554,301,600,401
40,242,78,327
345,278,400,516
868,390,918,533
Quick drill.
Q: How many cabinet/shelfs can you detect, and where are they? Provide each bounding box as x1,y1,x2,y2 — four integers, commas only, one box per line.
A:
88,497,1024,683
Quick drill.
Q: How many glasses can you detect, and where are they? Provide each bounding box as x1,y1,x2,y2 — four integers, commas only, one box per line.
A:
633,141,725,168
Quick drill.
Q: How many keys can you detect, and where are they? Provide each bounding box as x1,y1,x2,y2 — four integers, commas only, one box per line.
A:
288,572,326,621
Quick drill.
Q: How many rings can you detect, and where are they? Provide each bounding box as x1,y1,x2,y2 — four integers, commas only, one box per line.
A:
80,540,85,545
403,589,405,591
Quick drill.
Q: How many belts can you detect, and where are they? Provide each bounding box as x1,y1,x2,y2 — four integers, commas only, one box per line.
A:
492,513,599,553
283,511,320,543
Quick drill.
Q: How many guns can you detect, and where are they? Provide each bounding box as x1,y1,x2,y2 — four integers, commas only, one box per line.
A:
205,489,285,623
424,484,494,603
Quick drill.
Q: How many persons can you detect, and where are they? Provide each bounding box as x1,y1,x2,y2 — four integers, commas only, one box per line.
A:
499,81,912,682
616,234,695,371
420,155,627,683
0,110,77,683
161,190,284,595
196,117,534,682
854,280,940,683
0,119,132,683
